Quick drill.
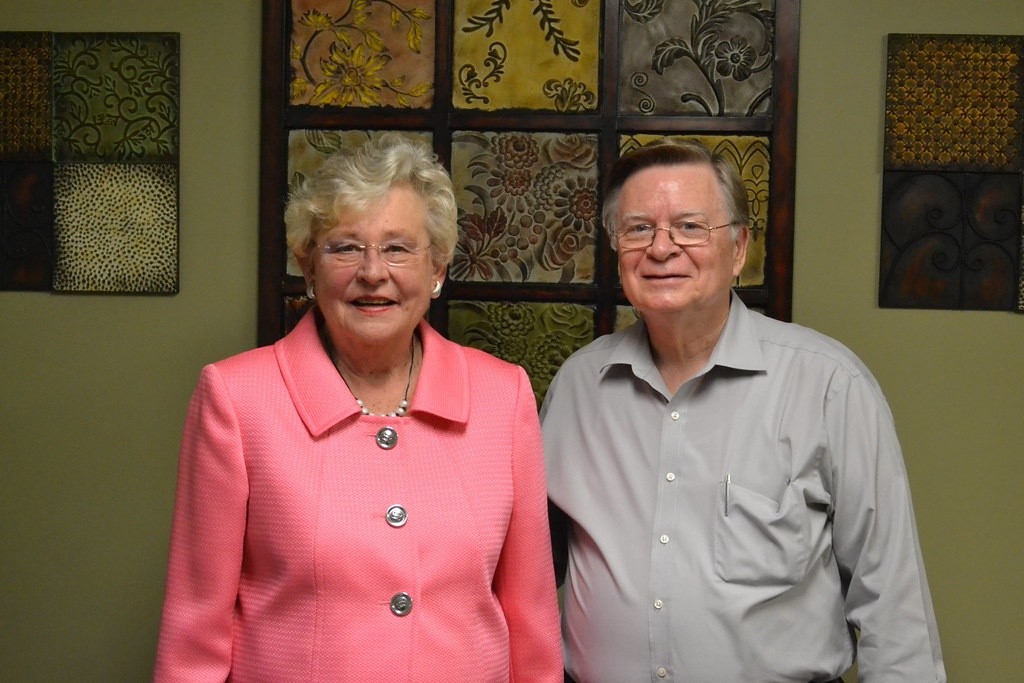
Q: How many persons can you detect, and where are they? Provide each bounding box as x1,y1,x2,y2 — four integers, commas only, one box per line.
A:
536,132,946,683
149,133,562,683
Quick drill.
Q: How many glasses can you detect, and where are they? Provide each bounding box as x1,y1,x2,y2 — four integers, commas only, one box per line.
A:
612,220,733,250
309,240,432,268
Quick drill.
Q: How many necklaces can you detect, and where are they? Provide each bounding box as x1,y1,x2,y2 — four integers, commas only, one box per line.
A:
318,325,416,420
